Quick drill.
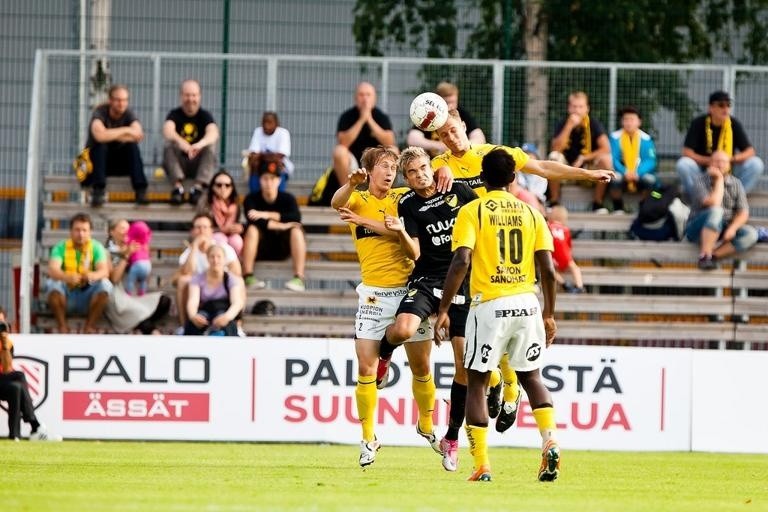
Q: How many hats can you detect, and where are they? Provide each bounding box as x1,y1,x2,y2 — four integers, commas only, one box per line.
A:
709,92,731,103
258,160,280,179
127,222,152,245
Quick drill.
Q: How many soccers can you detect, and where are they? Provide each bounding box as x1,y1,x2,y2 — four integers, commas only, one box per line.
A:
410,92,448,132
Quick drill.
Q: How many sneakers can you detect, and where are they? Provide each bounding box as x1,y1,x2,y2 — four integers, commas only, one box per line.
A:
89,194,109,207
134,195,152,206
170,187,184,207
188,191,201,206
359,353,561,481
244,276,265,290
695,254,718,271
284,276,306,292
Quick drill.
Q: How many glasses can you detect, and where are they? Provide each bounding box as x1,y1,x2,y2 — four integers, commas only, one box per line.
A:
215,182,233,189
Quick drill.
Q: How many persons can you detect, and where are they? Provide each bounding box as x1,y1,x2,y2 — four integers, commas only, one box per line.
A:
333,80,398,183
372,145,472,473
431,109,619,434
609,106,657,215
45,169,248,337
79,84,154,206
405,79,483,152
330,146,444,466
242,161,309,294
547,91,612,216
433,148,560,482
674,89,764,269
0,305,64,443
163,79,219,205
241,110,297,190
